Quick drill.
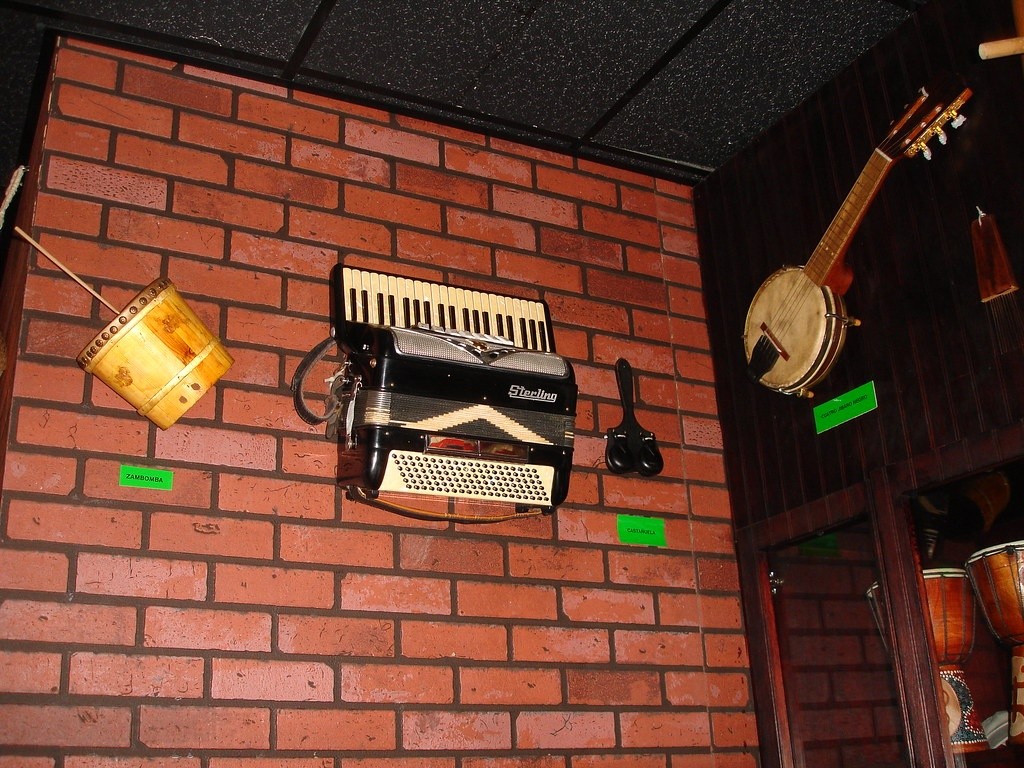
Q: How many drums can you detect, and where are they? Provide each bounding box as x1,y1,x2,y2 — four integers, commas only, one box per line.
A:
962,540,1024,748
865,566,994,759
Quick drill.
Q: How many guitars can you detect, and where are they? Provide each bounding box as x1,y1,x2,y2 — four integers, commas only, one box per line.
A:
740,76,973,401
